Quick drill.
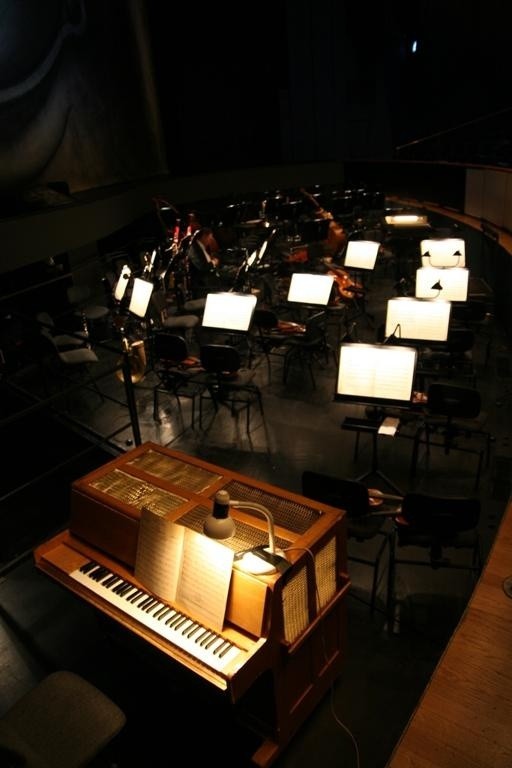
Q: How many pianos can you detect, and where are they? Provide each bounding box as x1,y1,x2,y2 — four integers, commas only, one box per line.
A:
34,441,351,768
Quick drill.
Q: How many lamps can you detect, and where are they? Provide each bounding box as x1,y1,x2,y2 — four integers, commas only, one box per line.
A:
202,488,288,576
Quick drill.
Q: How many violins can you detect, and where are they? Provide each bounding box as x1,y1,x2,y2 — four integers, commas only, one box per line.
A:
320,257,363,300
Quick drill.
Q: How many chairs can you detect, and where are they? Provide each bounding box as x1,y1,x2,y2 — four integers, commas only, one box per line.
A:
300,468,483,636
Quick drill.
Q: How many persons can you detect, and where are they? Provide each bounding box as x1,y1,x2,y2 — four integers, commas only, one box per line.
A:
185,227,221,293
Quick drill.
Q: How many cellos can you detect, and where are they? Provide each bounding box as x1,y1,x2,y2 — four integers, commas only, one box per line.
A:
297,187,346,250
